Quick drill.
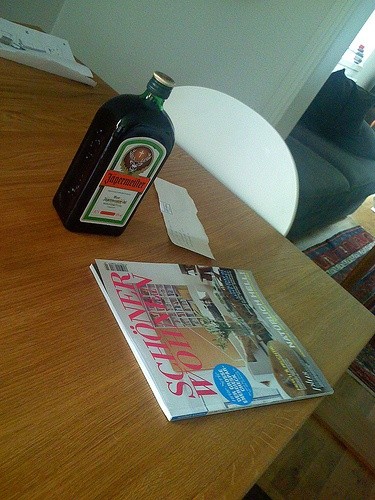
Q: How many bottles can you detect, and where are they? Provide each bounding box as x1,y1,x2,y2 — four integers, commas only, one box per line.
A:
53,70,176,238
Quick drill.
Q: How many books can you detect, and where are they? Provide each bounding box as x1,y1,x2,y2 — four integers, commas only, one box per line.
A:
90,257,336,423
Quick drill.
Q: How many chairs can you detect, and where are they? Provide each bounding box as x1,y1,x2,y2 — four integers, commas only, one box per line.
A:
162,87,300,237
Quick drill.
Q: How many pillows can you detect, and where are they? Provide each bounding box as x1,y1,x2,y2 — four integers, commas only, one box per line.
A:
303,67,375,142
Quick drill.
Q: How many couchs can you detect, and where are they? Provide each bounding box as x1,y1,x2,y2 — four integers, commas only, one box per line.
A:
283,120,375,241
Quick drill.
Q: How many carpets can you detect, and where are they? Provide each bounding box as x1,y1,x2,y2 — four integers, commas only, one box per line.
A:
302,225,375,400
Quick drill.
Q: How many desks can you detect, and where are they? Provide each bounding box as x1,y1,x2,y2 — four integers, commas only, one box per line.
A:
0,20,375,500
